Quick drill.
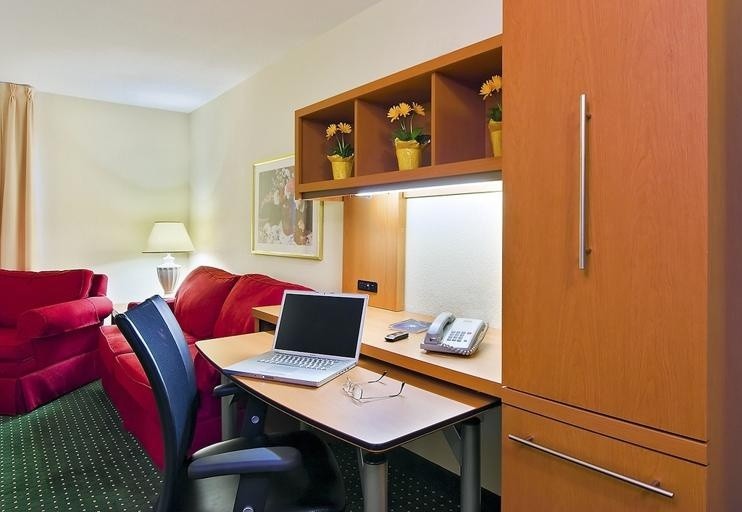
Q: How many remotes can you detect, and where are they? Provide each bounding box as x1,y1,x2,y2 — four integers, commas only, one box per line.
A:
384,330,410,342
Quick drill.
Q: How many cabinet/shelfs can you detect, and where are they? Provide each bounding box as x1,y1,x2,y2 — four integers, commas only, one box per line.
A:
501,31,742,512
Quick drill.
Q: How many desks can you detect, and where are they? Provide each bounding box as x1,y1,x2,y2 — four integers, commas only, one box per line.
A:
195,290,512,512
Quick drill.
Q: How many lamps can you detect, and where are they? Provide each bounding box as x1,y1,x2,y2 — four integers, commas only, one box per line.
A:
142,221,197,300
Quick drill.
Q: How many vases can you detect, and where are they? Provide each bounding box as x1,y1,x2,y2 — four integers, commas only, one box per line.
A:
485,119,502,157
326,154,353,179
388,138,430,170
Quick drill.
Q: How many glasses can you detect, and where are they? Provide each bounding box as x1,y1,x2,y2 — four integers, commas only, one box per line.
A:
340,371,406,403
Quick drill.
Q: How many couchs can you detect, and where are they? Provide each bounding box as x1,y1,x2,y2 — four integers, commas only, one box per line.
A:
98,265,335,471
0,265,113,419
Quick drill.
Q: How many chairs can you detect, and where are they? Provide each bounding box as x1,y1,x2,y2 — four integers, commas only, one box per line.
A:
106,294,348,512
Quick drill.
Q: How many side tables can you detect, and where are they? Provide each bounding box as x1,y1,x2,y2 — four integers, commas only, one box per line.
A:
105,306,128,327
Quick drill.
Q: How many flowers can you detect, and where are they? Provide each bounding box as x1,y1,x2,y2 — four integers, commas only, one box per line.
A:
382,101,429,146
319,121,354,158
477,75,505,124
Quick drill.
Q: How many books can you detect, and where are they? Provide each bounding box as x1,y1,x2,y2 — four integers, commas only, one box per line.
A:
388,317,433,334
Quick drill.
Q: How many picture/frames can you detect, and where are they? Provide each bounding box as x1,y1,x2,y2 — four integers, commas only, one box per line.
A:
245,154,325,262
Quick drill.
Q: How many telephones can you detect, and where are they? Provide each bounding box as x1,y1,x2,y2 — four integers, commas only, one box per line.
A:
420,313,489,357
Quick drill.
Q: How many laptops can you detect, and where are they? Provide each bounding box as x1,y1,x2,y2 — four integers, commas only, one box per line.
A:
221,289,370,388
